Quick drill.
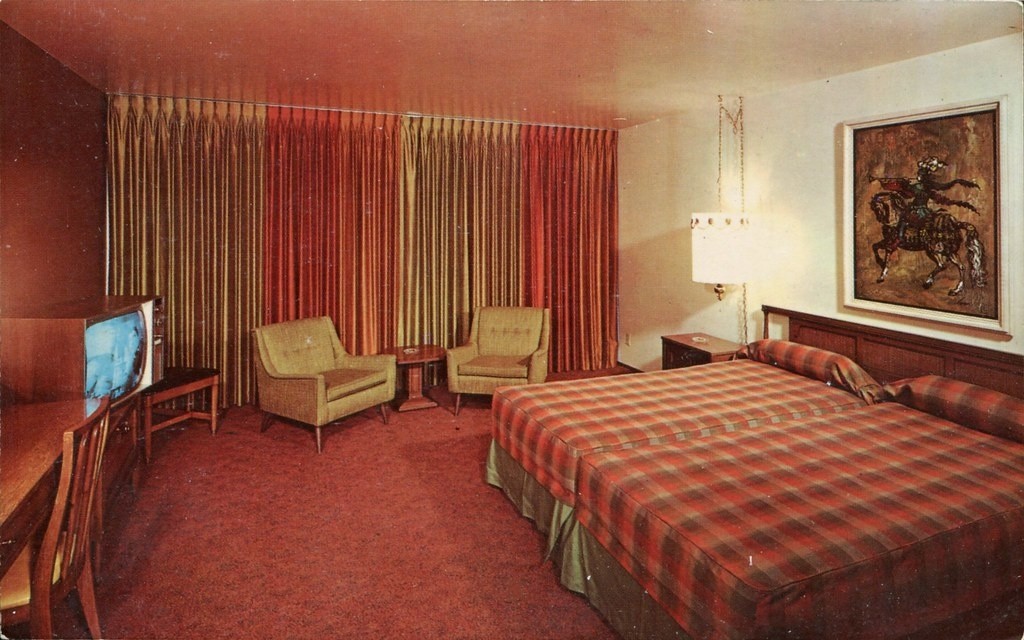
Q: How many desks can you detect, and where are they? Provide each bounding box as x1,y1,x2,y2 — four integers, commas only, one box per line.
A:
0,398,103,579
141,367,220,466
382,345,447,412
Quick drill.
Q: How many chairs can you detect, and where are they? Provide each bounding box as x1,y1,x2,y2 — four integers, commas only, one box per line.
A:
0,394,112,640
250,315,395,454
446,307,549,415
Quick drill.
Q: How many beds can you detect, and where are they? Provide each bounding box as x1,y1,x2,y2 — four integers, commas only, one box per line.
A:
552,335,1024,640
486,304,913,568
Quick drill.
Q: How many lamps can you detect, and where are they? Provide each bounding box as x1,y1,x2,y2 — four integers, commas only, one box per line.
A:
692,95,751,344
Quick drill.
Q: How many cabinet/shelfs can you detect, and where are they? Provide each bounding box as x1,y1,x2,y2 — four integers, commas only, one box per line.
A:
103,394,141,512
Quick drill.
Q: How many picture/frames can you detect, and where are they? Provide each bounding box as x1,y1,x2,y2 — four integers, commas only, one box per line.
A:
843,93,1013,337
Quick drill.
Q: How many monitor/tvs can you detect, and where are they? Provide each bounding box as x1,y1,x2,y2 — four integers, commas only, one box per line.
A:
0,295,165,409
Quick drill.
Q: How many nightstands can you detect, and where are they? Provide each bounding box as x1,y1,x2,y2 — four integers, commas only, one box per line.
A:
661,333,744,370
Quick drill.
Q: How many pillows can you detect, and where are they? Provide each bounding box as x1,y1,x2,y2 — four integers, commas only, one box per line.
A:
880,374,1024,443
733,339,882,405
